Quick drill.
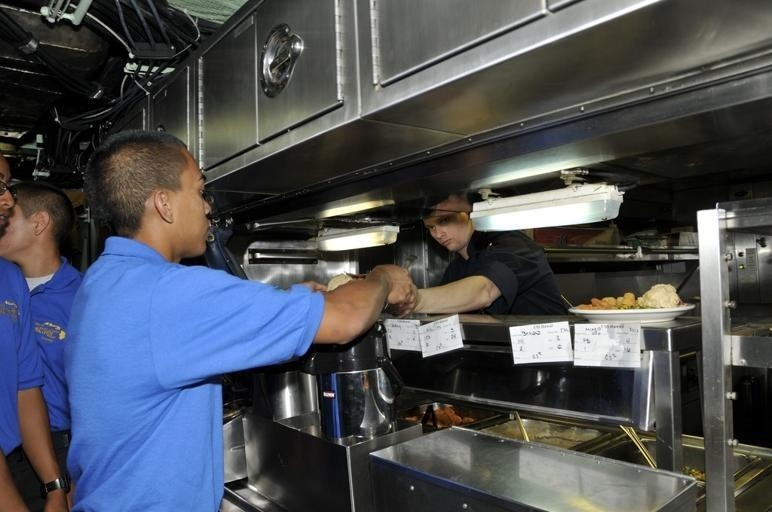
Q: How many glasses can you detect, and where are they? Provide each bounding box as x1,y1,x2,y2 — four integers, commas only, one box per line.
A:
1,181,17,197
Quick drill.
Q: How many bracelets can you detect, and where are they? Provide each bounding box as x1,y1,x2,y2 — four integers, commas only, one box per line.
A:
41,473,71,496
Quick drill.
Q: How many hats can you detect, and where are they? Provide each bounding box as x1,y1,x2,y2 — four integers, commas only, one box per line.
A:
420,194,473,215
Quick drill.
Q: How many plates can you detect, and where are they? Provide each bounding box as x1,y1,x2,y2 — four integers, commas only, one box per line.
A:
567,303,698,326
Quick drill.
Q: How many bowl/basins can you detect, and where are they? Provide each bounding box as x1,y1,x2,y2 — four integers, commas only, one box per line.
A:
625,234,667,247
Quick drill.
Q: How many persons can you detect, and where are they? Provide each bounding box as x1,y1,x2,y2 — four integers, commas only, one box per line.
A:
386,189,569,318
63,128,413,512
0,153,70,512
0,182,87,435
